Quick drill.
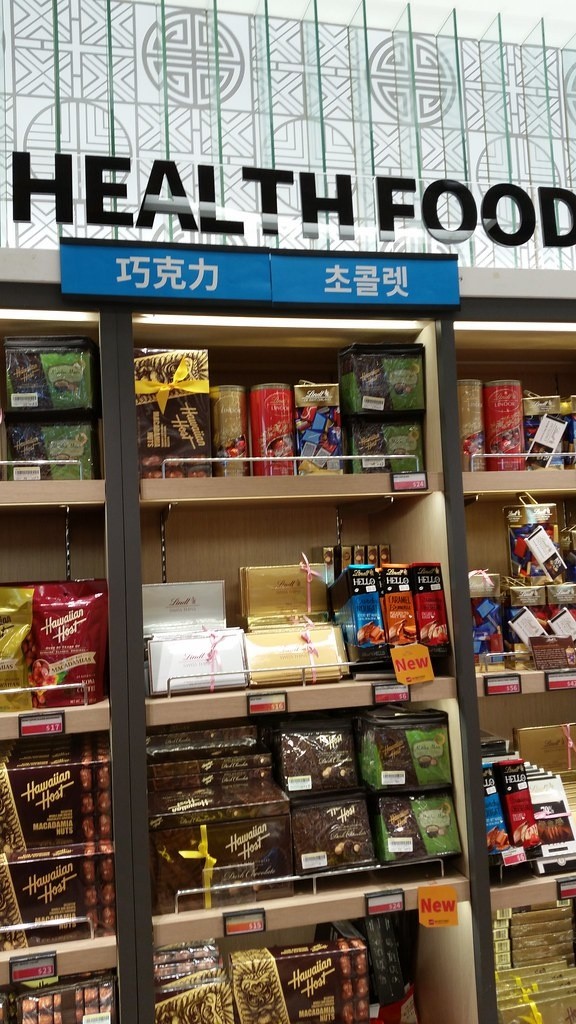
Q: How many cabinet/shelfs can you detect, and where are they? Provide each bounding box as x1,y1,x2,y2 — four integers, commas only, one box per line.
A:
116,294,498,1024
0,280,138,1024
454,298,576,1024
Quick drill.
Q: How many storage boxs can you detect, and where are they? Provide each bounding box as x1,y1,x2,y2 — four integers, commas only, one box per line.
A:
141,544,453,698
0,732,119,953
228,937,372,1024
